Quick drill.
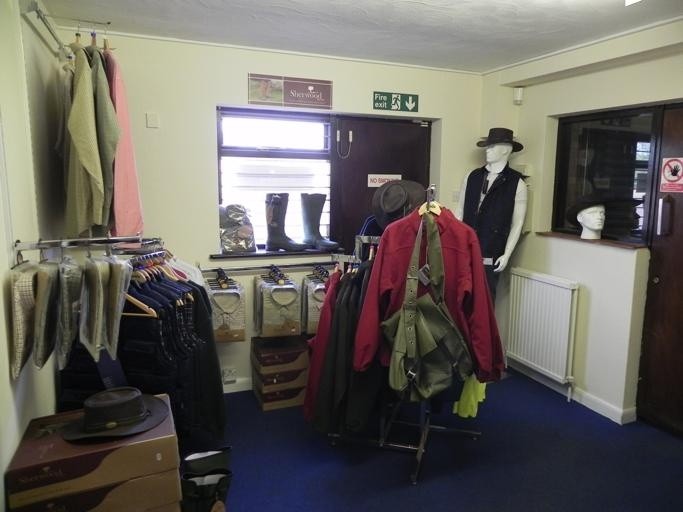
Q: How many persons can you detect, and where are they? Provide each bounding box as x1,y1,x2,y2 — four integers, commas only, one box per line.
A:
565,192,613,240
454,127,529,386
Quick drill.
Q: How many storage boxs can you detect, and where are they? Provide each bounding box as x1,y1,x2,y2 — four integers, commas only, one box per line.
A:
3,394,183,512
251,338,310,412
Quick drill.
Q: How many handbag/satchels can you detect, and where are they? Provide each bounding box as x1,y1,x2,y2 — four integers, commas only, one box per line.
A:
380,293,473,402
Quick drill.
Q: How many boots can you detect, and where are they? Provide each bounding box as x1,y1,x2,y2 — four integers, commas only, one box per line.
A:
180,445,233,512
266,193,307,252
301,194,339,250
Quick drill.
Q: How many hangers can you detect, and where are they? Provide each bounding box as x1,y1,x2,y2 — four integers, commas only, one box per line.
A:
333,235,374,274
71,249,193,319
418,182,442,223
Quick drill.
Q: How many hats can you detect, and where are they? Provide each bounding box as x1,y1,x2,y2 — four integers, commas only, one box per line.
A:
477,128,523,152
567,195,604,224
372,180,426,231
63,387,169,440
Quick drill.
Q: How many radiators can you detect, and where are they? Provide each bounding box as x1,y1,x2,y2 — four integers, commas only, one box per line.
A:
501,266,580,403
